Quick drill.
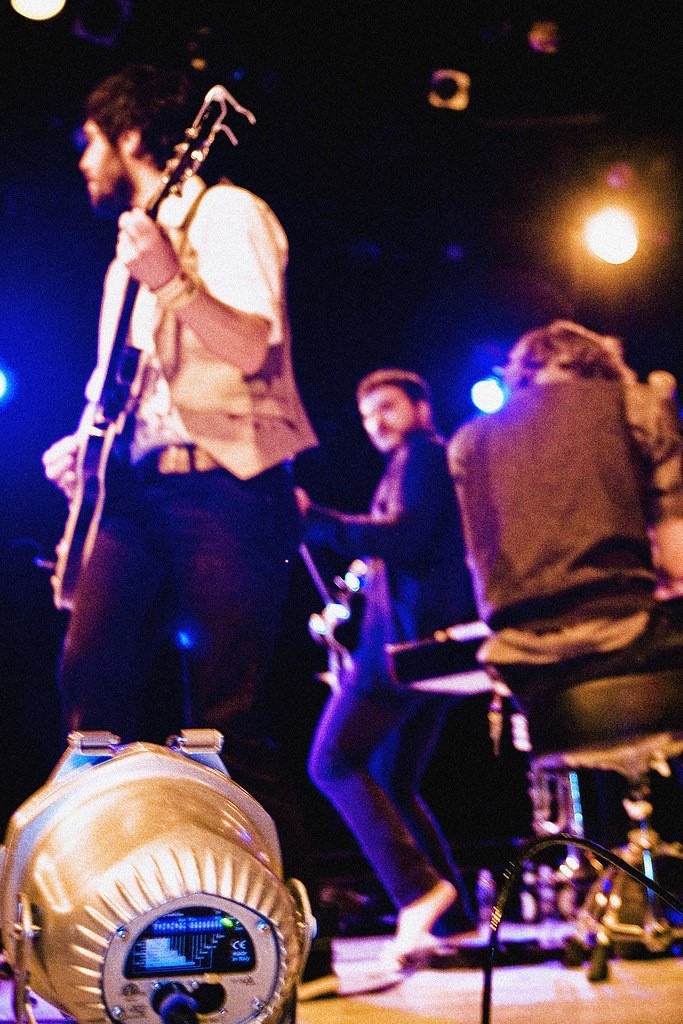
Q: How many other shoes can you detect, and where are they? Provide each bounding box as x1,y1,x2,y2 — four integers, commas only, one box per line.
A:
362,926,462,975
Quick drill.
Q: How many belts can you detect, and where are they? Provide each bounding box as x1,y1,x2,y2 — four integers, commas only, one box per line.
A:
150,445,219,474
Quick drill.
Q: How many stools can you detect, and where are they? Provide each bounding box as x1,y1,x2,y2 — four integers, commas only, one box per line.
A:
383,622,604,970
523,668,683,982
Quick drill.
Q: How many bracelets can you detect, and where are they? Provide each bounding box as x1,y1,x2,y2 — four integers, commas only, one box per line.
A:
147,268,200,311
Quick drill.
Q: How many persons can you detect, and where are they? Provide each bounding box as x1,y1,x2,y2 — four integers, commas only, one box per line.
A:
294,370,481,973
446,319,683,711
41,67,340,1002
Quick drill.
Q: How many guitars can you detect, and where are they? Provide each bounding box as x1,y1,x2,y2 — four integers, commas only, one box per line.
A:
52,85,257,611
281,469,366,692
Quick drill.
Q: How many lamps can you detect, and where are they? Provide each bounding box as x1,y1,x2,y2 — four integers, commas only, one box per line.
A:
0,729,316,1024
